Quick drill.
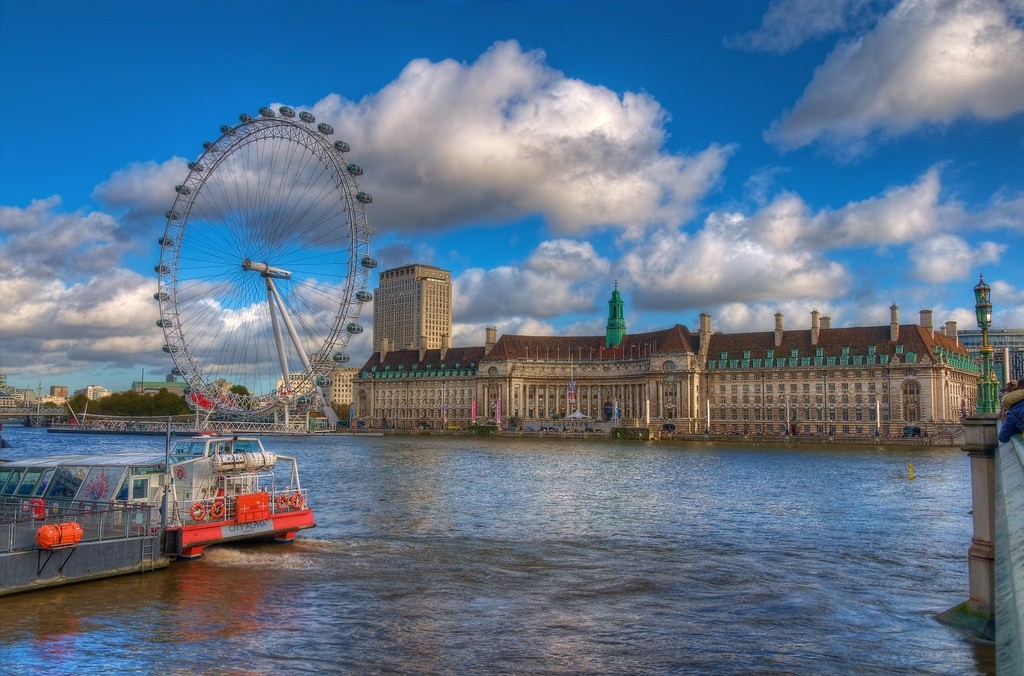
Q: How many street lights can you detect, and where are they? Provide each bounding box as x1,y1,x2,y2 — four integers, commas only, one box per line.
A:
974,273,1000,414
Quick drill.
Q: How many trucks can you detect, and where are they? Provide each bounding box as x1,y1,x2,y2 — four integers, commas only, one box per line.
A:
417,424,431,429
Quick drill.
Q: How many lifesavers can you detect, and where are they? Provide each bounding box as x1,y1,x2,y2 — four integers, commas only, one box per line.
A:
290,492,304,509
27,498,46,521
209,501,226,521
276,495,289,511
190,502,208,522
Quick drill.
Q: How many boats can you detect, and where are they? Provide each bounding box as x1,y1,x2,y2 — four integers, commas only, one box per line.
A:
0,419,317,558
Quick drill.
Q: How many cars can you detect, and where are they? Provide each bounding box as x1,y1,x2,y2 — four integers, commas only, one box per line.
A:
446,425,460,430
480,420,500,426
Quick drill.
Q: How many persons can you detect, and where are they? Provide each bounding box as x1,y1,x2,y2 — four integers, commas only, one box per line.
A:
997,380,1024,443
